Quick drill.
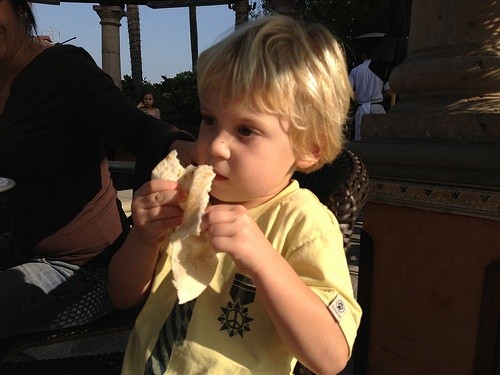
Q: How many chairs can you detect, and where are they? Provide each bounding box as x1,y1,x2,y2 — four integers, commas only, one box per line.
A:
291,136,370,303
0,119,203,374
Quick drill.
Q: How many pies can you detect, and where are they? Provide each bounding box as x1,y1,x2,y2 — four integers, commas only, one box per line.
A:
151,149,220,304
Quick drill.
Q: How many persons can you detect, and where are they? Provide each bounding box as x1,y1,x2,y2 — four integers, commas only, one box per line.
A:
348,48,392,140
137,91,160,120
107,15,362,375
0,0,197,355
